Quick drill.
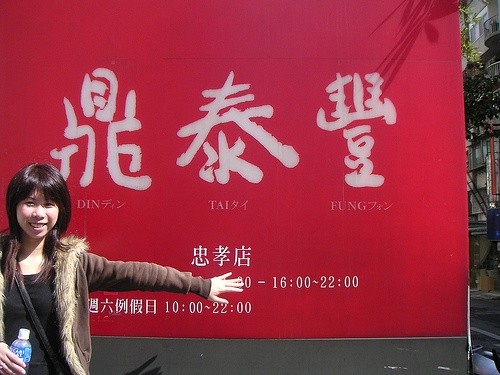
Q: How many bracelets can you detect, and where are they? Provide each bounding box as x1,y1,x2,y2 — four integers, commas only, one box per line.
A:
0,341,6,344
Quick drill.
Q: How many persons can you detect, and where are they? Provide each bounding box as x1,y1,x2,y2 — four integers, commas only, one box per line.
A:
0,161,244,375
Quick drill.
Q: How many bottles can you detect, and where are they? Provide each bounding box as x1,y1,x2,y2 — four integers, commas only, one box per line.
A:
9,328,32,374
